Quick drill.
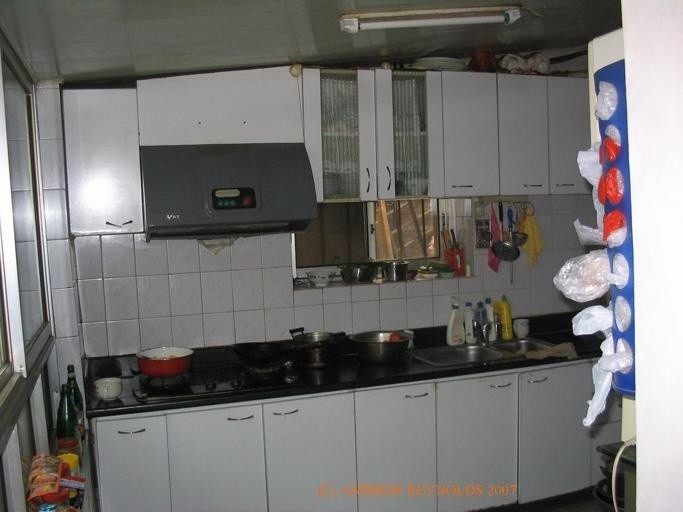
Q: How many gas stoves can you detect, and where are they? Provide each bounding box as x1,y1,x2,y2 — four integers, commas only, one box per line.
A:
130,364,304,402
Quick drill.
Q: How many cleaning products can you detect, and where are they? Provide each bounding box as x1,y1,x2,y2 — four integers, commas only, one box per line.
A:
476,301,486,320
446,302,475,346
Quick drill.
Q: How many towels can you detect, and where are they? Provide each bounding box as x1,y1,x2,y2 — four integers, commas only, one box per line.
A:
519,207,545,264
488,203,501,273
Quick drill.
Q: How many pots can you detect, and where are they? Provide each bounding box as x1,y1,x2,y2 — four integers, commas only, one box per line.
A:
226,338,326,369
134,347,196,379
290,326,346,371
336,260,379,285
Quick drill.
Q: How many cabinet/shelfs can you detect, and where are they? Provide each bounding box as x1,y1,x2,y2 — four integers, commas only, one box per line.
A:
91,357,636,512
137,65,592,203
62,89,146,235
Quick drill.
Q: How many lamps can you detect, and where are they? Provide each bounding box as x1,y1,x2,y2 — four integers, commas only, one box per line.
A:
339,6,522,34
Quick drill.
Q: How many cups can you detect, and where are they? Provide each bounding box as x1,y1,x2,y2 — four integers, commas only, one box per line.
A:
471,52,491,72
92,376,126,402
511,318,532,339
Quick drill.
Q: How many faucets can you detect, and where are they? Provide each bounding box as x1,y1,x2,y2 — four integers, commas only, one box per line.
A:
471,319,500,346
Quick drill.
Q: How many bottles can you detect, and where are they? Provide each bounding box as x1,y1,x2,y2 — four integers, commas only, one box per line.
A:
55,363,85,468
446,293,514,348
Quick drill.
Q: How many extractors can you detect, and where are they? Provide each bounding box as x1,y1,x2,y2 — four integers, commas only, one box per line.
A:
135,141,321,244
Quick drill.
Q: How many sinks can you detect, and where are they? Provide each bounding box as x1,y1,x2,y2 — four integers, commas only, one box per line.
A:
413,346,504,367
494,337,559,354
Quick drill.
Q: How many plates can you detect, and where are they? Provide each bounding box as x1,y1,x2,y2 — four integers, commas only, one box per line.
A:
412,56,466,70
418,273,438,278
438,271,454,278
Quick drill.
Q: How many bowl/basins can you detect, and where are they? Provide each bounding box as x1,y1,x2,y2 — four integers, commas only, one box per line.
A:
344,330,416,368
379,261,409,282
306,271,334,287
